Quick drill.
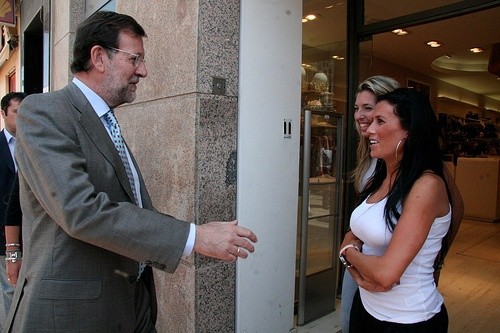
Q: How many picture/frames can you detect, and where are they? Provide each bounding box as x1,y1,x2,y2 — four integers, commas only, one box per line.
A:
405,78,431,104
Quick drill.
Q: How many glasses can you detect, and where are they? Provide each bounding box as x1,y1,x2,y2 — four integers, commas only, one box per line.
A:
102,45,148,66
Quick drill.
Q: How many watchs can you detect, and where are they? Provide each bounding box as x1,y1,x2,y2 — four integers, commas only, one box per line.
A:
339,244,359,268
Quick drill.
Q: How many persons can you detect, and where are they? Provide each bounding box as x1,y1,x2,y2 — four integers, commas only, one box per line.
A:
14,9,257,333
310,133,335,209
0,92,33,333
338,76,500,333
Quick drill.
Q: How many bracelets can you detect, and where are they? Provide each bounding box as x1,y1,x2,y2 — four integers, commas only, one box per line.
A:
4,249,22,263
5,244,20,247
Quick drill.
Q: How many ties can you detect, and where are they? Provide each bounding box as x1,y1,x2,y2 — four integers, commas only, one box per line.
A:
103,110,146,281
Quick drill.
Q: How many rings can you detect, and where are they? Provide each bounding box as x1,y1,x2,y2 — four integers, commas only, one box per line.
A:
8,278,10,281
235,248,240,256
